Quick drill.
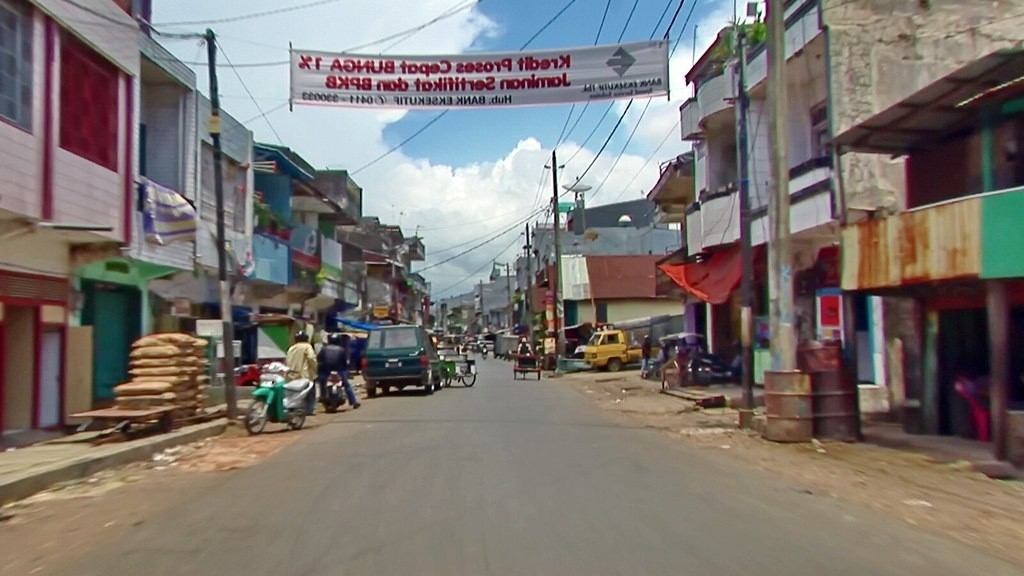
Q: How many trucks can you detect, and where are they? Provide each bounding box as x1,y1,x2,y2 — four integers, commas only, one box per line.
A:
584,325,659,372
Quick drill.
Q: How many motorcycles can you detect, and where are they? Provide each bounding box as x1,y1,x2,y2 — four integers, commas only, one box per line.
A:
321,369,347,413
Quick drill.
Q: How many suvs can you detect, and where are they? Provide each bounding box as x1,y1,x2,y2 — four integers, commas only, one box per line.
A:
360,324,443,399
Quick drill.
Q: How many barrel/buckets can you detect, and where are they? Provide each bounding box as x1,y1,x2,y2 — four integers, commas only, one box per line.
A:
762,372,812,441
857,384,880,414
798,339,862,442
1006,409,1024,464
904,399,921,433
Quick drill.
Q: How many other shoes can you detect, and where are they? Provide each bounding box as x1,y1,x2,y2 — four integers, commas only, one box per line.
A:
306,410,316,415
353,403,360,409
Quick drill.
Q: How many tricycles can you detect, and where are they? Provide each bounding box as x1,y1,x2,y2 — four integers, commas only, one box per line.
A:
513,354,542,381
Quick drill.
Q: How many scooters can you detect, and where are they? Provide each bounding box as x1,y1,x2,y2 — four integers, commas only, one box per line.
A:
244,360,317,437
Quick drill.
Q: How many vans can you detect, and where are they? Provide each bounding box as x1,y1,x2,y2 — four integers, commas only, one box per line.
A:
477,341,494,351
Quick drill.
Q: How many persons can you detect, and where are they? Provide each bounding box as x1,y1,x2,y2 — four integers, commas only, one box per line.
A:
284,332,318,417
517,337,534,356
317,334,360,409
640,335,669,378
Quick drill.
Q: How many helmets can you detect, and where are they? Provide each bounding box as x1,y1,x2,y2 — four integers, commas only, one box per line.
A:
328,333,341,343
295,330,308,341
